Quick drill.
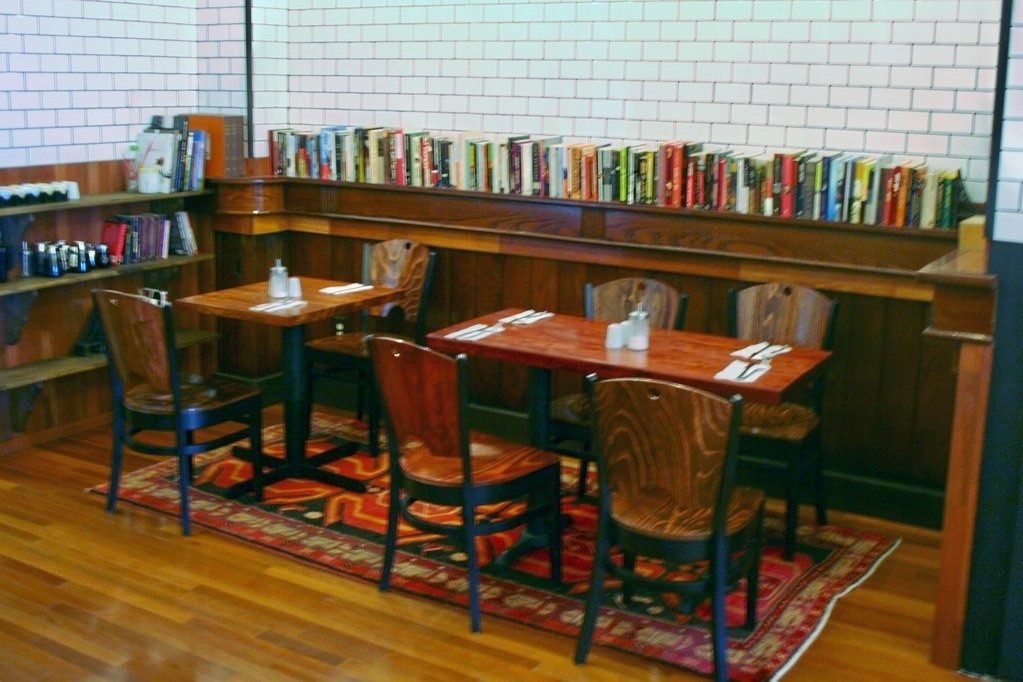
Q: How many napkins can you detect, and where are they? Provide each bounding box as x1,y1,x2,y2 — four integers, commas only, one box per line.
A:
714,358,772,384
248,297,308,313
443,322,505,341
318,281,374,296
498,308,556,325
729,340,793,361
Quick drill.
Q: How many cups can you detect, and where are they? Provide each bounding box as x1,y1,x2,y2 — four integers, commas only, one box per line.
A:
139,167,160,193
605,323,623,349
288,278,303,297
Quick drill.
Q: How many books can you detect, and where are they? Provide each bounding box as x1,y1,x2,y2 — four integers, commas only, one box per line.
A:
102,210,198,264
139,287,172,303
267,126,971,231
135,115,207,193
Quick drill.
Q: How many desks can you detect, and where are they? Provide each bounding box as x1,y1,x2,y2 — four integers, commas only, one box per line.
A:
174,274,408,501
424,308,836,618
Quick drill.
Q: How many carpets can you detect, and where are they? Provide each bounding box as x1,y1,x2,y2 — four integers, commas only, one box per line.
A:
84,412,907,682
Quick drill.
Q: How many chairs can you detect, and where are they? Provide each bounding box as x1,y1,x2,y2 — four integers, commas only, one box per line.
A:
363,333,566,634
724,279,843,563
303,236,438,458
89,288,263,537
573,370,767,682
549,276,690,501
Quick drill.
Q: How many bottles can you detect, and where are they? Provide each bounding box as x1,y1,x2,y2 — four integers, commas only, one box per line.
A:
269,266,288,297
628,311,650,350
127,145,140,193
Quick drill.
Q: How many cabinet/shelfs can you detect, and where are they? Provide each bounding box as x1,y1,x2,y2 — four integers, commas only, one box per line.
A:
0,190,222,391
